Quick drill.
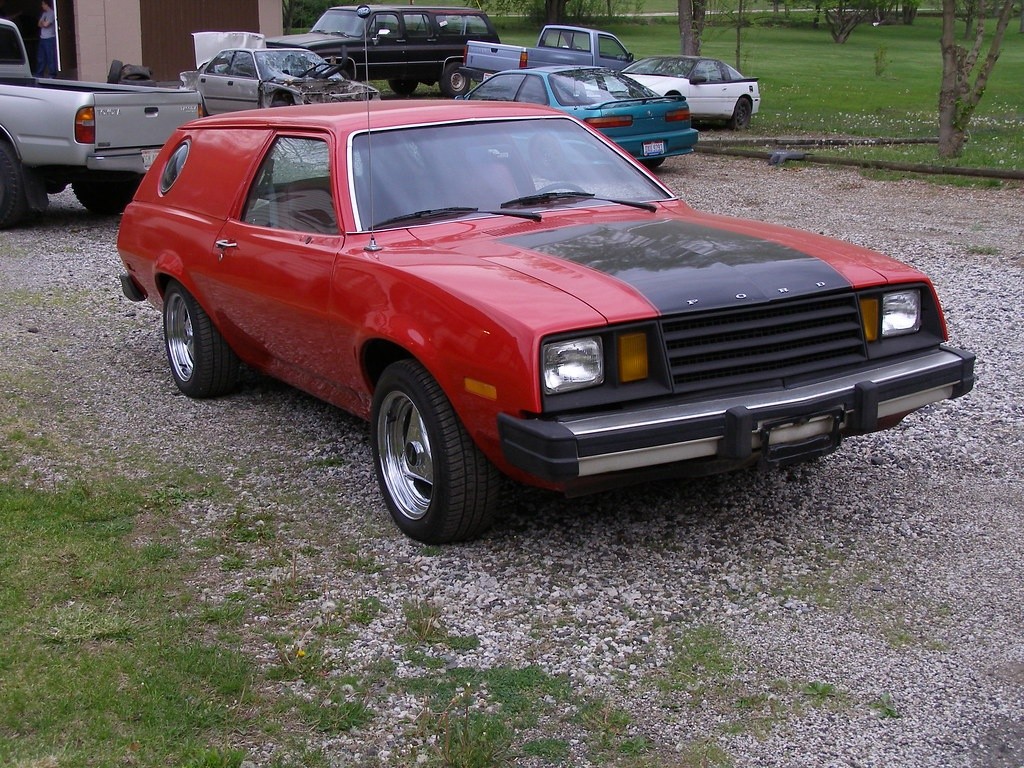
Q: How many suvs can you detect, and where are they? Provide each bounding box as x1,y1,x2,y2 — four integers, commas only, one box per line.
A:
264,4,501,98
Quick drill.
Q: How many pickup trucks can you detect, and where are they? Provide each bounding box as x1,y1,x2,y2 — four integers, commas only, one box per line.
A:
0,18,203,227
459,23,638,83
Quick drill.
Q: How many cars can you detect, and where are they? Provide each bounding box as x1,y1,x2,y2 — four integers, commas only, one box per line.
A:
179,31,382,115
454,65,699,170
115,92,979,545
621,53,761,131
